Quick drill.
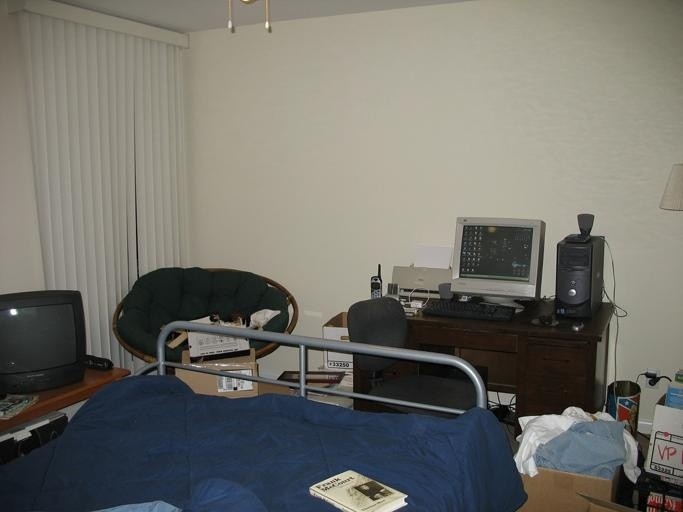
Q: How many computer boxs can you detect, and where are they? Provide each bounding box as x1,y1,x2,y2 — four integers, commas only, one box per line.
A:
554,234,605,321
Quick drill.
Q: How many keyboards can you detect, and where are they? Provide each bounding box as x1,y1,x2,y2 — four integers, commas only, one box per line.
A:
421,300,516,322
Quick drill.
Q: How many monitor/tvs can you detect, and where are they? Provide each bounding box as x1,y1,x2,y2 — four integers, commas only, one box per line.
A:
450,216,546,313
0,289,87,395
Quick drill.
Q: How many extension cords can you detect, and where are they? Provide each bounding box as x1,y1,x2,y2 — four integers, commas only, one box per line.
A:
502,413,517,425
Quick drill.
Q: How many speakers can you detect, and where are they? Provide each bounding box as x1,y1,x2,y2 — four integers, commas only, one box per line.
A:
564,213,595,244
438,282,454,300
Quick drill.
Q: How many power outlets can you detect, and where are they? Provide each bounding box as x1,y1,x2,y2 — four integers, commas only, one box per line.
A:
645,368,660,389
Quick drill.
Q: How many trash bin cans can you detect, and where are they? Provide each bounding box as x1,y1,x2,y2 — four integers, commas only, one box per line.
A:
606,380,641,437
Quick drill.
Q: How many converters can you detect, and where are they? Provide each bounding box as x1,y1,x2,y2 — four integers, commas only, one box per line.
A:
499,405,510,413
645,372,657,379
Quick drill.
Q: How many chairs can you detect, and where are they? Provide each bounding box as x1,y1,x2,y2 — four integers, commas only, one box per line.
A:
346,298,476,421
111,265,298,378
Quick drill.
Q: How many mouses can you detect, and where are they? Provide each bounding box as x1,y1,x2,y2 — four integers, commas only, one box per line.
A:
538,314,553,326
570,321,583,333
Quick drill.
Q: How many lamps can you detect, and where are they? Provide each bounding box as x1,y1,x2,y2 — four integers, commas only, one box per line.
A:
659,165,683,211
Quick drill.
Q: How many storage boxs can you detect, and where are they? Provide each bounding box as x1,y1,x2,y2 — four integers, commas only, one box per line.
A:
516,463,620,512
636,471,683,512
175,348,258,399
643,393,683,481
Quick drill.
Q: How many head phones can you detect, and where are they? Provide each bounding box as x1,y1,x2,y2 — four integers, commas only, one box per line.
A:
491,408,506,419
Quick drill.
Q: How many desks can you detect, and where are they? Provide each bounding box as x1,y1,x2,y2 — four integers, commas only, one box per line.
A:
352,299,615,440
0,368,130,435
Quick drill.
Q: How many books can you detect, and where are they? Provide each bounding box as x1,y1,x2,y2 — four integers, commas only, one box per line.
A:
184,310,251,363
0,393,39,421
307,467,408,512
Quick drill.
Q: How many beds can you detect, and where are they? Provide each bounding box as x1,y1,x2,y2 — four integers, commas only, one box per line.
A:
0,321,528,512
